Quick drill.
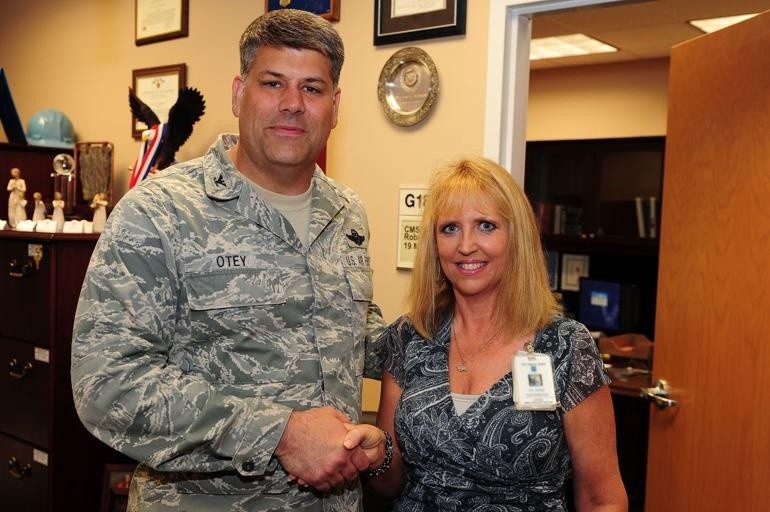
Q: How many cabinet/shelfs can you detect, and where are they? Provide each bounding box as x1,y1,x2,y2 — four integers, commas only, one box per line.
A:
519,133,666,345
0,141,114,512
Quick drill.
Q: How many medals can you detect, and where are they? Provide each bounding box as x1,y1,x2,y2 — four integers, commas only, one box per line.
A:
376,47,442,129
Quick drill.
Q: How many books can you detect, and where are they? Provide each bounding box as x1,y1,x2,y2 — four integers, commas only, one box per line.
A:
532,198,585,237
636,195,656,238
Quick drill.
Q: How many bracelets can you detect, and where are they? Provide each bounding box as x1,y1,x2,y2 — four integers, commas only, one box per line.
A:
367,426,395,479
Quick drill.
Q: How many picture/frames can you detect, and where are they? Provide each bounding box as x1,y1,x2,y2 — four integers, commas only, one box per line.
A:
135,0,188,46
130,63,187,138
373,1,466,45
264,0,341,25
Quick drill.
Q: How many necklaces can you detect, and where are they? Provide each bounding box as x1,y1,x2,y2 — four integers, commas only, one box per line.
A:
444,305,520,373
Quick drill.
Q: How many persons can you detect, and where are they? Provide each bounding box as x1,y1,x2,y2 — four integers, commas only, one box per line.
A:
69,7,385,512
343,157,629,511
6,167,28,228
